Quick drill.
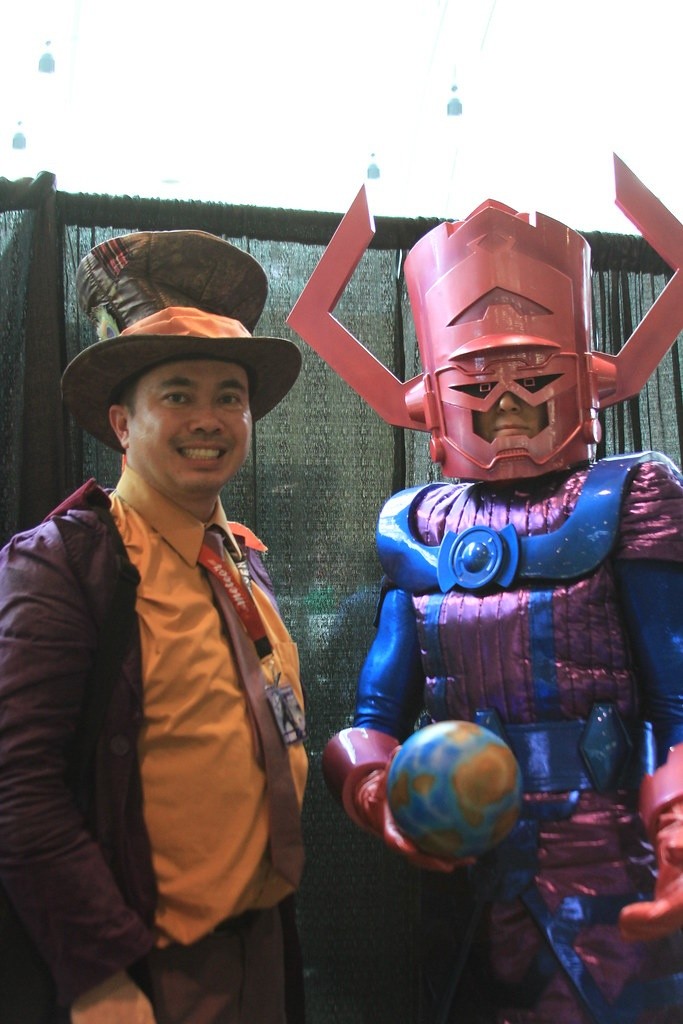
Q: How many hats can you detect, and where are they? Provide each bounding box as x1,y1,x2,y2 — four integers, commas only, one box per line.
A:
60,229,302,454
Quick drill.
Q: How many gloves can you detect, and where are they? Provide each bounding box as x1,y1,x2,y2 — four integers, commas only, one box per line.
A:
355,745,479,875
619,802,683,946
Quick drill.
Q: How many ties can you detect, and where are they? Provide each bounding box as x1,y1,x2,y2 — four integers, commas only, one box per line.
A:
201,525,306,891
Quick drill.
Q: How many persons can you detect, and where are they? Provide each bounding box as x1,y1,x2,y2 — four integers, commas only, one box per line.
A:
347,305,683,1023
0,326,308,1023
